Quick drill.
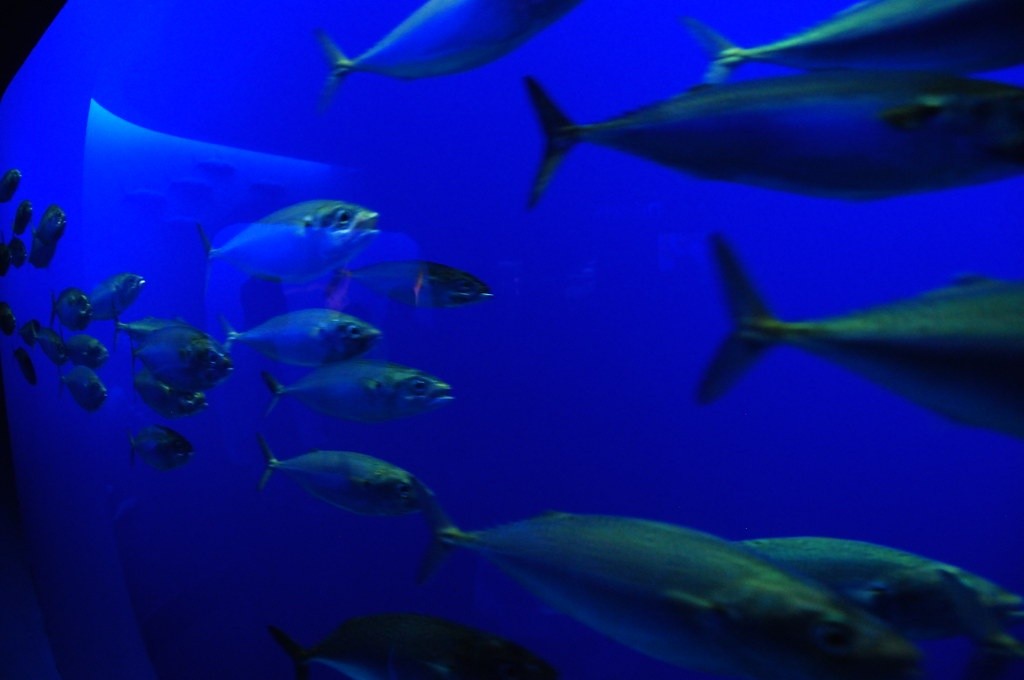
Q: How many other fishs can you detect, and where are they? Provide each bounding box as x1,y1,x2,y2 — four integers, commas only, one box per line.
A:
1,1,1023,680
